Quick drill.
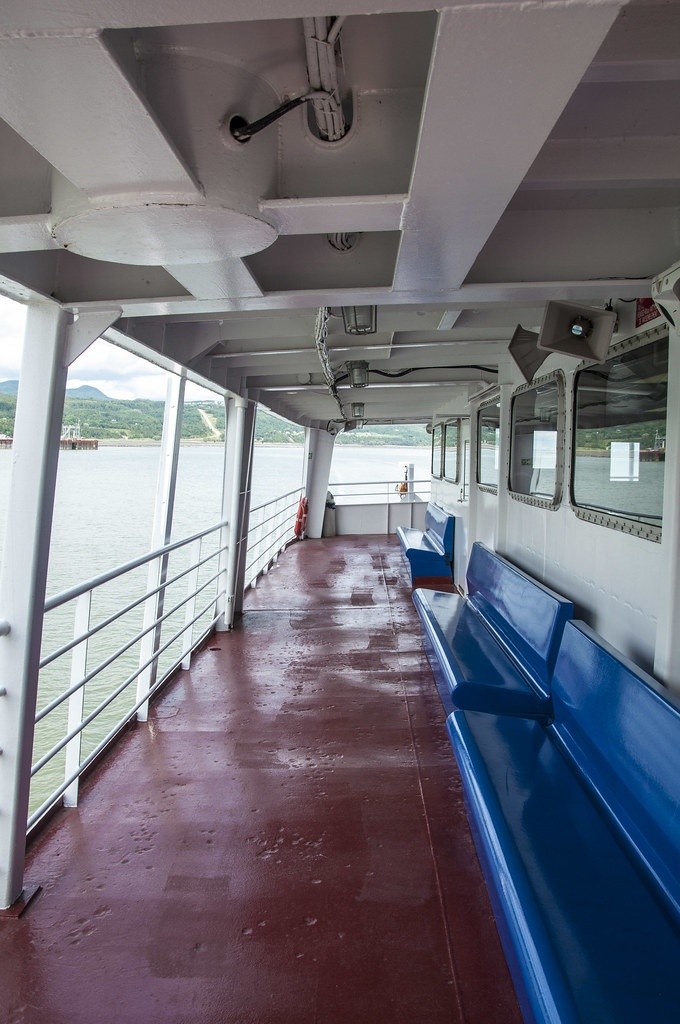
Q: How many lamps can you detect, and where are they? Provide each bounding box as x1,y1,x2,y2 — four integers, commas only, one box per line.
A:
351,402,365,417
345,360,370,388
355,419,364,429
342,304,378,337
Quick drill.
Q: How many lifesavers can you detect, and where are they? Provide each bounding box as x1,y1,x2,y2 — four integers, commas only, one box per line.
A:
294,496,308,536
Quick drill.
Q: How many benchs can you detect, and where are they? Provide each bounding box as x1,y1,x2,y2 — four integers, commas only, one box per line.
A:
446,618,680,1024
396,501,456,585
410,541,574,722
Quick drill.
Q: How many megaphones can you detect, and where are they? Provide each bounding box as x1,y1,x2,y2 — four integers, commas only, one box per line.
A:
507,323,553,386
537,300,617,365
